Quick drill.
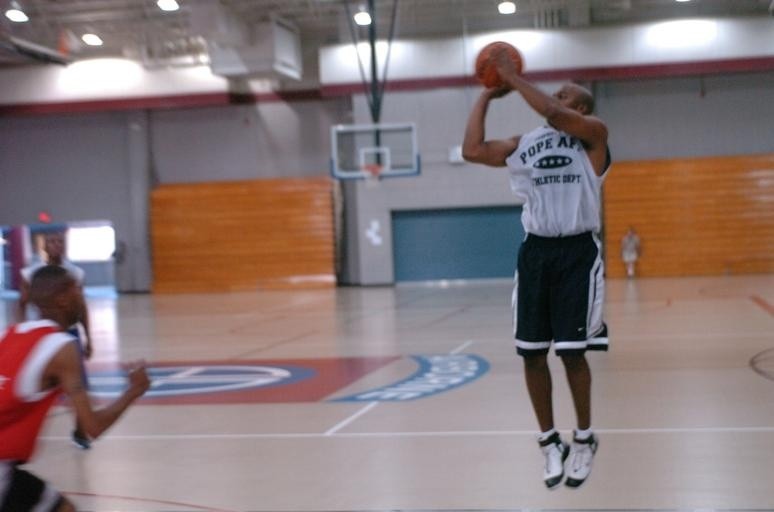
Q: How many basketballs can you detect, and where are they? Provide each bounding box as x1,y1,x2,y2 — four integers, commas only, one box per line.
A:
477,42,523,88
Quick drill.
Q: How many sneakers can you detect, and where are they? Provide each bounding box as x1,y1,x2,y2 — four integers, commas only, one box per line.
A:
537,431,569,488
70,432,90,449
565,426,598,488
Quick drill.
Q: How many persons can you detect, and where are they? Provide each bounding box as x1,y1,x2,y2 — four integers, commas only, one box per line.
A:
620,228,640,278
463,47,611,489
0,265,151,512
11,230,94,450
